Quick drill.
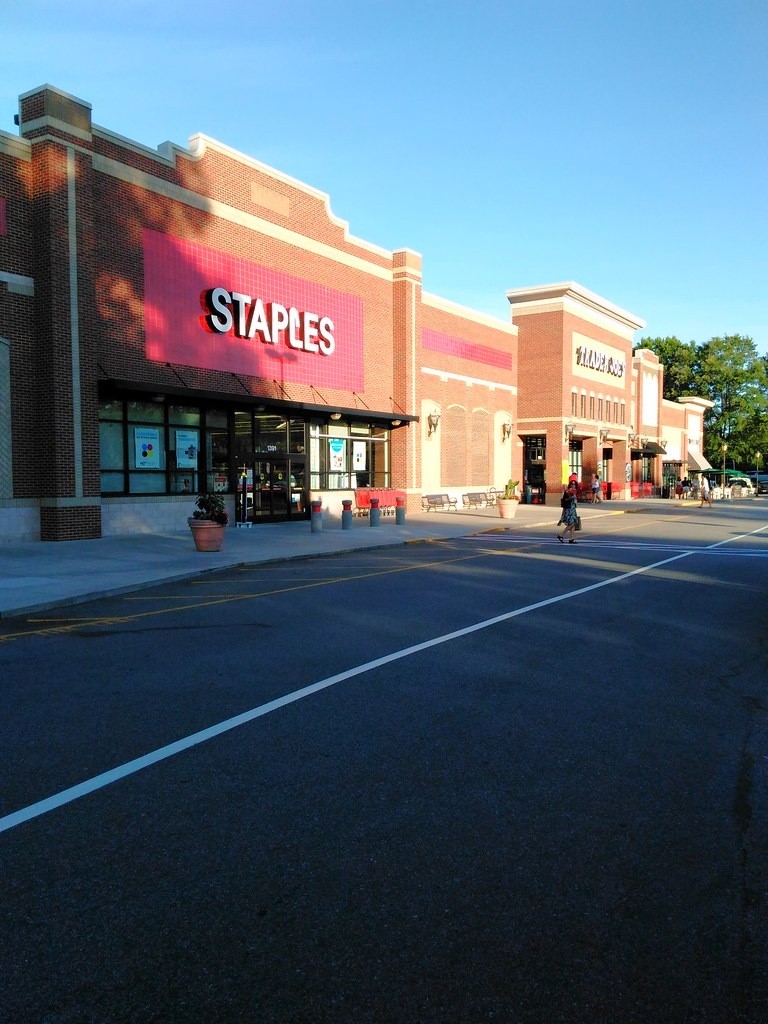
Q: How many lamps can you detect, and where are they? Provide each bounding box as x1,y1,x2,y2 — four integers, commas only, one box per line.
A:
661,441,668,449
330,413,341,421
503,420,512,442
641,435,648,449
391,420,401,426
599,425,610,447
152,394,165,402
428,409,441,437
565,419,576,442
628,430,636,448
252,405,265,412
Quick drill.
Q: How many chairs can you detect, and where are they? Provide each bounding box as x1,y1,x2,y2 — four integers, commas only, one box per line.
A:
713,487,746,500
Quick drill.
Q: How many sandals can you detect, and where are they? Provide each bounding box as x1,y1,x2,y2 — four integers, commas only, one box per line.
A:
557,535,563,543
569,539,578,544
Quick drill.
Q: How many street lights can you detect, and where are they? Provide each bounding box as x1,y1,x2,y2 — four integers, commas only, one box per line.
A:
754,451,759,497
721,445,728,498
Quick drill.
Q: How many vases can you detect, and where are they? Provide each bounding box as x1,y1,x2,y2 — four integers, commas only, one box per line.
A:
187,520,228,551
496,500,519,519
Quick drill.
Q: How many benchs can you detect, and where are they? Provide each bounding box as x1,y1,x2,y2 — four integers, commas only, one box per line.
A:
462,492,495,509
422,494,457,512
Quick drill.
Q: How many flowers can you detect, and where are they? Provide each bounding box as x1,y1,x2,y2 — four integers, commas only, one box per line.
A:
193,488,229,527
496,479,520,500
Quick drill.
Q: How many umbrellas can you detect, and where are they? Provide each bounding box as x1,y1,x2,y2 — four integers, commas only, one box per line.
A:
694,468,750,486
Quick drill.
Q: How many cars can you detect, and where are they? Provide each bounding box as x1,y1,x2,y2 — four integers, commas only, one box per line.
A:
747,471,768,494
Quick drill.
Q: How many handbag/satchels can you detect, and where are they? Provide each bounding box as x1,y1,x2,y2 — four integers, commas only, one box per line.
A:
561,491,571,509
569,517,582,530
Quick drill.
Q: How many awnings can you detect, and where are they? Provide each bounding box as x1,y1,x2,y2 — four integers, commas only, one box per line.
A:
644,441,667,454
98,379,419,430
631,449,655,455
689,451,712,471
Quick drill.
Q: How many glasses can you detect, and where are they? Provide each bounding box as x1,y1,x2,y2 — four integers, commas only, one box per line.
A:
571,483,575,485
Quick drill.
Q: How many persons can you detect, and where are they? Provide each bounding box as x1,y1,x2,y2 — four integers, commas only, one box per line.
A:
590,474,604,504
675,474,717,509
557,473,580,543
